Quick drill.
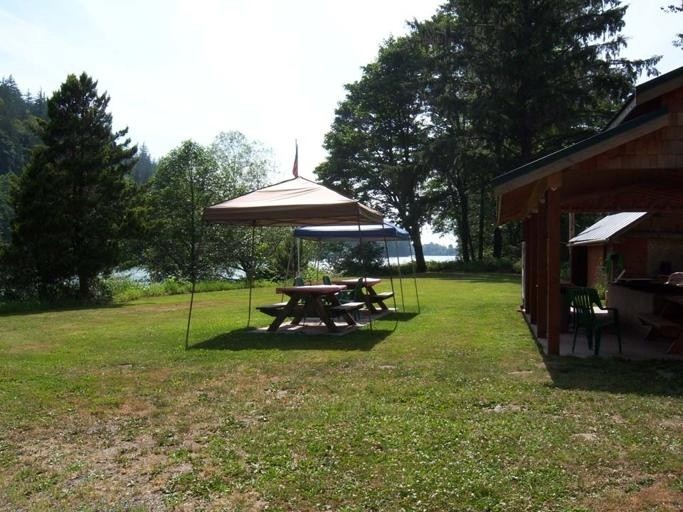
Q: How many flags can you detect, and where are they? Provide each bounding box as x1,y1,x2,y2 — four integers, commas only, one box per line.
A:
293,146,298,177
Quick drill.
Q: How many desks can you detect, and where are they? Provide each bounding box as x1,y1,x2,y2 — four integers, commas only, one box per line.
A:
275,285,347,332
334,277,389,312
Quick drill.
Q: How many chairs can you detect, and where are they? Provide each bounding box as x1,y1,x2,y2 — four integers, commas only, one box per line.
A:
292,277,303,285
322,275,331,284
341,277,365,300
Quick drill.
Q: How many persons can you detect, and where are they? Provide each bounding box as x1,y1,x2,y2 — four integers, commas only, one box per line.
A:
607,255,623,281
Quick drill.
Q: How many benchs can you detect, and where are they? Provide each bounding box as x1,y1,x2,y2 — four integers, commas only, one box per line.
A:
355,290,394,313
255,302,364,333
